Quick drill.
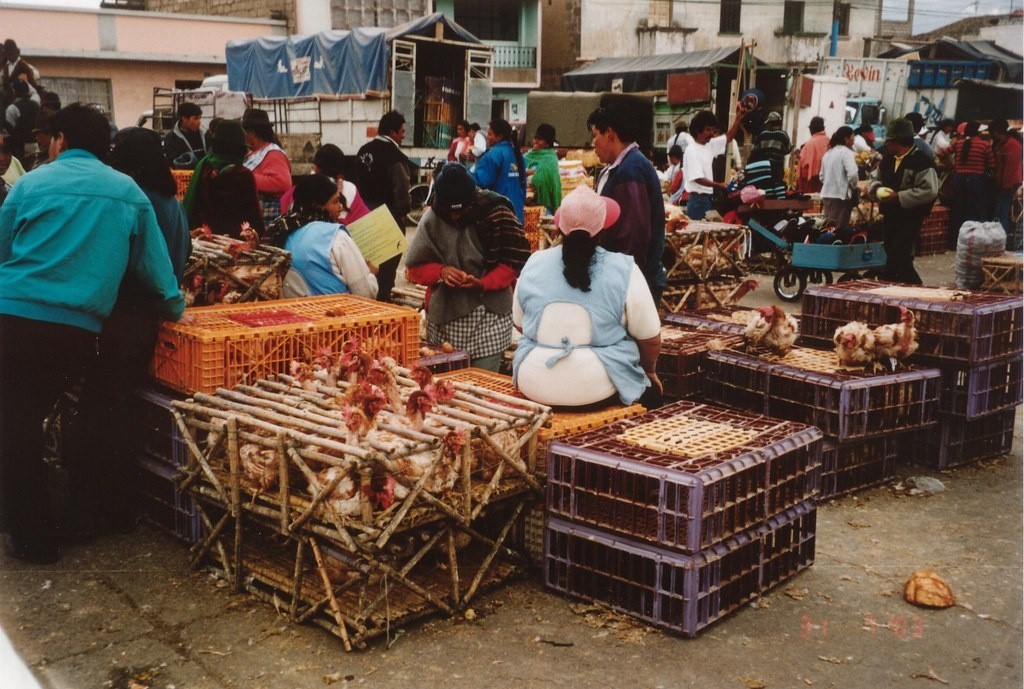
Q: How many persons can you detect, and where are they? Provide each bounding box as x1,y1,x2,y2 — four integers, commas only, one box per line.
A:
1,40,1024,562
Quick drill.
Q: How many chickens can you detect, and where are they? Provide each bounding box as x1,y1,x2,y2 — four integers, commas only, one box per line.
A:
181,223,287,308
205,335,529,518
743,305,799,361
871,305,919,373
723,278,759,305
834,320,876,367
664,202,744,276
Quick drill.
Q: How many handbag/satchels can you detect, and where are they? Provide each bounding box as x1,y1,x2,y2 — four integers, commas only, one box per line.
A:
943,173,976,205
839,188,861,207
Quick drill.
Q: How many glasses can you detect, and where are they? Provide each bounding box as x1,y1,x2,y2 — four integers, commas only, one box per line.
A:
533,135,548,142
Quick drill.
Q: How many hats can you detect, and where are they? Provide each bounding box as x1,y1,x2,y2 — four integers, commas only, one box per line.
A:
763,111,782,124
554,185,621,238
985,117,1009,131
302,173,338,210
29,109,57,134
435,160,476,210
883,117,916,140
208,120,248,148
533,123,557,143
956,122,968,136
242,109,274,130
867,131,876,142
675,120,689,129
666,145,684,154
4,38,20,54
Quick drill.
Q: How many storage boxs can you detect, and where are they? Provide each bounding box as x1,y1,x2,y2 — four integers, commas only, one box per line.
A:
116,168,1024,652
